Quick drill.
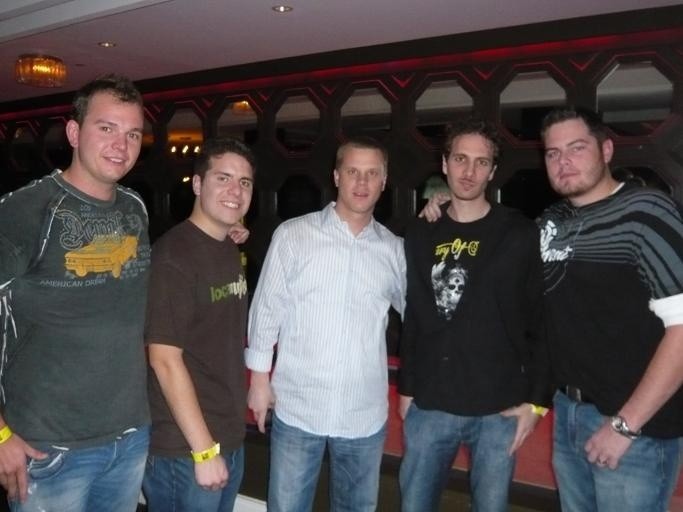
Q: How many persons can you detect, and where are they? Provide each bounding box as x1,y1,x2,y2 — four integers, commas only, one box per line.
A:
245,135,409,510
146,137,254,510
387,108,555,510
415,106,682,510
0,71,251,510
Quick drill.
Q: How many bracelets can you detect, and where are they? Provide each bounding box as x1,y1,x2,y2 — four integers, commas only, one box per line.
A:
0,426,13,445
531,403,550,416
187,443,226,460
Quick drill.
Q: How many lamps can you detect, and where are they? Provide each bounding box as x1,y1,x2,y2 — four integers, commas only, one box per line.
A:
14,50,67,91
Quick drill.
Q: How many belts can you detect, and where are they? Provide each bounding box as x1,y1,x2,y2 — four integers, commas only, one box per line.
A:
554,383,595,405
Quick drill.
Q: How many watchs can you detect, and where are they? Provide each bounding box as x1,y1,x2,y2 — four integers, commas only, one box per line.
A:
610,413,644,441
